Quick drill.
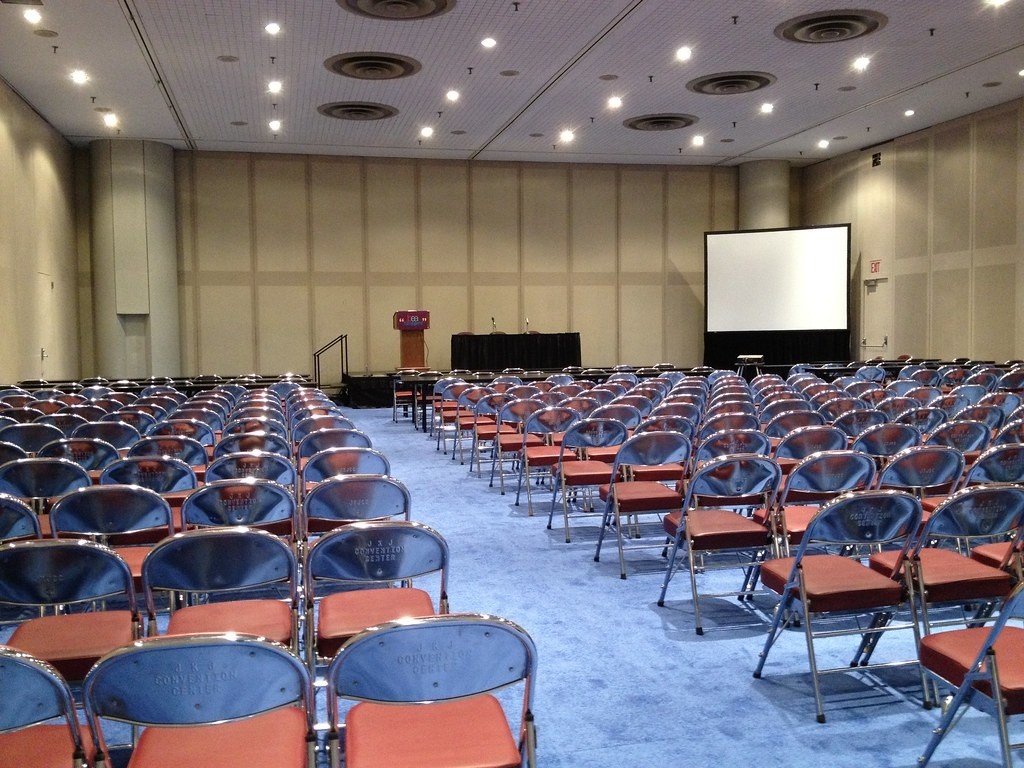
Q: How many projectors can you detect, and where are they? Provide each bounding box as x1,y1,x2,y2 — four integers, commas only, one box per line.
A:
737,355,764,363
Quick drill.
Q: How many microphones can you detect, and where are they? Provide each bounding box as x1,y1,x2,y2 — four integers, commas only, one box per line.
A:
525,317,529,324
492,317,496,327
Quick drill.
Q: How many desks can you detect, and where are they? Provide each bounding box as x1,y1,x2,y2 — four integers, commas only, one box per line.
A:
451,333,581,372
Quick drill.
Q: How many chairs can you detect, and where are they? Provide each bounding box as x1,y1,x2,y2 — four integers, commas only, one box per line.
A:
0,353,1024,767
